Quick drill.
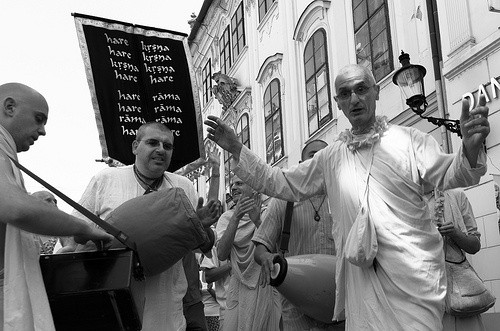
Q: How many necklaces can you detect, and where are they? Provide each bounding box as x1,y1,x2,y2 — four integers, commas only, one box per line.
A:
308,192,328,222
134,163,164,191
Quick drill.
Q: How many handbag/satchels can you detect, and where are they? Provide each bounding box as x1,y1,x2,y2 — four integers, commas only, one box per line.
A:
445,254,497,318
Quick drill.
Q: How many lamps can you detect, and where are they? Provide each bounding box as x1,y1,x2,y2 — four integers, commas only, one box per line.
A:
392,49,488,152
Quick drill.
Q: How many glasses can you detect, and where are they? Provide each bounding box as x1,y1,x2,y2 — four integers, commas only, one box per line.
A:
144,139,174,151
335,85,370,100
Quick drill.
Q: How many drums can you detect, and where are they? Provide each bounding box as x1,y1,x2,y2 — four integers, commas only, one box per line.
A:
270,254,345,323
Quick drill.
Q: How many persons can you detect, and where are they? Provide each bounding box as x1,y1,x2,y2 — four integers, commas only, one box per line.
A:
203,65,491,331
195,174,282,331
27,191,75,255
426,189,484,331
71,123,218,331
0,83,115,331
251,140,345,331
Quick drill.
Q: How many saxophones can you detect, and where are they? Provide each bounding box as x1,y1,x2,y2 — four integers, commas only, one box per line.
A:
432,190,445,227
